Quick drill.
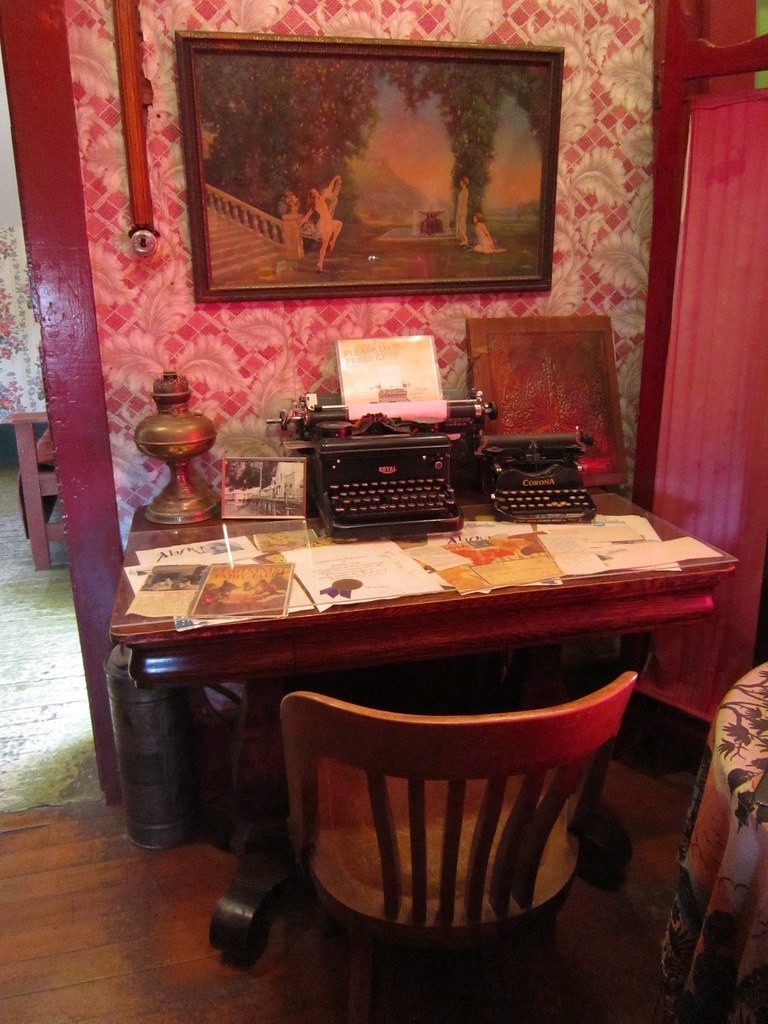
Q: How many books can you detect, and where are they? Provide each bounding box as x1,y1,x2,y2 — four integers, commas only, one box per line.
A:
190,562,295,619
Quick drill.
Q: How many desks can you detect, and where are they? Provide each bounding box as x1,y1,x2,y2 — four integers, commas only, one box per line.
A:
106,493,739,958
661,661,768,1023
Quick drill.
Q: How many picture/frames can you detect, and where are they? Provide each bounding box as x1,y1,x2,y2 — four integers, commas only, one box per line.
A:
220,456,307,520
176,34,566,304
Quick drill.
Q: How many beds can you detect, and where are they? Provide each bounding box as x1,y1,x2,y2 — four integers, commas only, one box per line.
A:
10,412,69,570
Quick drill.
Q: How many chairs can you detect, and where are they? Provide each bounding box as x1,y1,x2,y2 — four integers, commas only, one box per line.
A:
277,667,636,1024
